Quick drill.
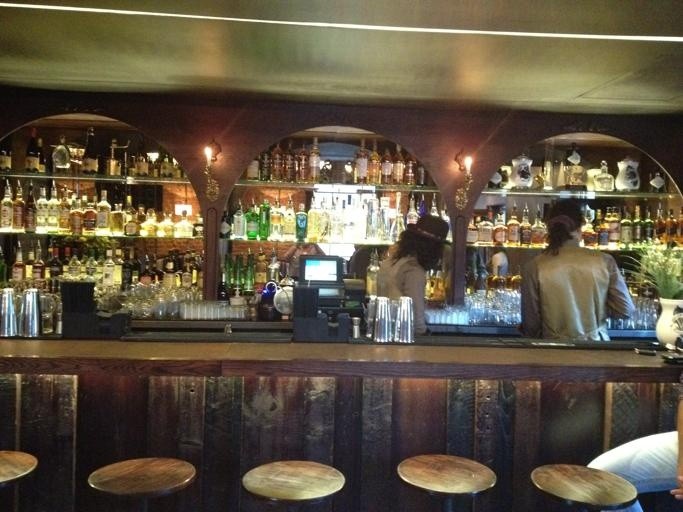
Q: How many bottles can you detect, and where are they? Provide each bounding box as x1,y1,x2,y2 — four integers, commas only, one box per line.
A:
466,143,683,250
221,134,441,290
365,249,391,298
0,125,203,288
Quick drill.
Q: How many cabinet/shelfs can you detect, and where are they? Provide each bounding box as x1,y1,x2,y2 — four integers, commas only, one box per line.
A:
0,117,204,311
464,139,682,299
220,130,452,302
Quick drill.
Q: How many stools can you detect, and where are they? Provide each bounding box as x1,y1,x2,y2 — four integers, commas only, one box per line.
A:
0,450,38,512
87,458,198,512
397,453,497,512
242,460,346,511
531,463,638,511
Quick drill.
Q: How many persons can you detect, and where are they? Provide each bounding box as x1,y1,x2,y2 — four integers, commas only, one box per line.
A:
567,399,683,512
377,214,450,335
515,196,636,340
284,244,325,281
467,240,509,290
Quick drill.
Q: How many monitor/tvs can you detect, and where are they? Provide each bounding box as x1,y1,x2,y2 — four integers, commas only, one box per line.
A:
299,255,342,285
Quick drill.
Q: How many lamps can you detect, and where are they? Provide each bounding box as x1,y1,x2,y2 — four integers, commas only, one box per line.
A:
204,146,222,202
455,156,476,210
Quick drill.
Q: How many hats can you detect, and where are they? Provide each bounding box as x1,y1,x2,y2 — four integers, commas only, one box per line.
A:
407,214,452,246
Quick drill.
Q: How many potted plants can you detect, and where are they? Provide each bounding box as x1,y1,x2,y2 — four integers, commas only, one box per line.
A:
620,246,683,350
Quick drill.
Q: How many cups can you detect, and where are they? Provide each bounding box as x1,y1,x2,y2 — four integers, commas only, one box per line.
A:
462,274,521,325
364,295,414,345
124,282,248,324
608,294,655,332
1,278,62,340
